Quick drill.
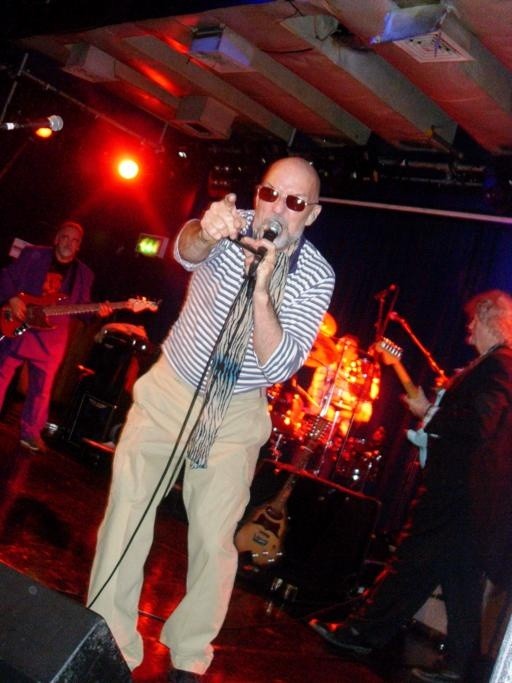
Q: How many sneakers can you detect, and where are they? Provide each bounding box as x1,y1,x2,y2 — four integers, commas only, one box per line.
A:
20,434,48,452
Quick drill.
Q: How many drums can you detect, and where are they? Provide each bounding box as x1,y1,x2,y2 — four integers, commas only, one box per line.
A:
266,384,305,435
335,437,381,482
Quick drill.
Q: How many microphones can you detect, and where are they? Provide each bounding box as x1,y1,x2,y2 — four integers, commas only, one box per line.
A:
248,220,283,276
4,113,64,132
373,283,397,300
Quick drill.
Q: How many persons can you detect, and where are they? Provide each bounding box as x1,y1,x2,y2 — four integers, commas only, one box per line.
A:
308,288,512,683
1,220,113,453
84,155,338,680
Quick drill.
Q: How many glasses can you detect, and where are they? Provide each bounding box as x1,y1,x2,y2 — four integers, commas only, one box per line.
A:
258,185,318,213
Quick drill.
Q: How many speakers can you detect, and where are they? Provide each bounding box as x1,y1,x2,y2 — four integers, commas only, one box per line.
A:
86,335,148,404
0,561,134,683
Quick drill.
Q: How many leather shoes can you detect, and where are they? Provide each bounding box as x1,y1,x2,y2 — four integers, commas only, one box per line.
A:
411,664,478,683
310,617,377,654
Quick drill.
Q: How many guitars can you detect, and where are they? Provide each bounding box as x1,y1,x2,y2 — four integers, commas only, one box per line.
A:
0,292,159,337
376,336,428,468
234,415,334,568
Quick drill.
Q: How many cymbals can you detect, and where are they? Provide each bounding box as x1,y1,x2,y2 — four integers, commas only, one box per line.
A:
317,312,337,337
304,332,337,368
